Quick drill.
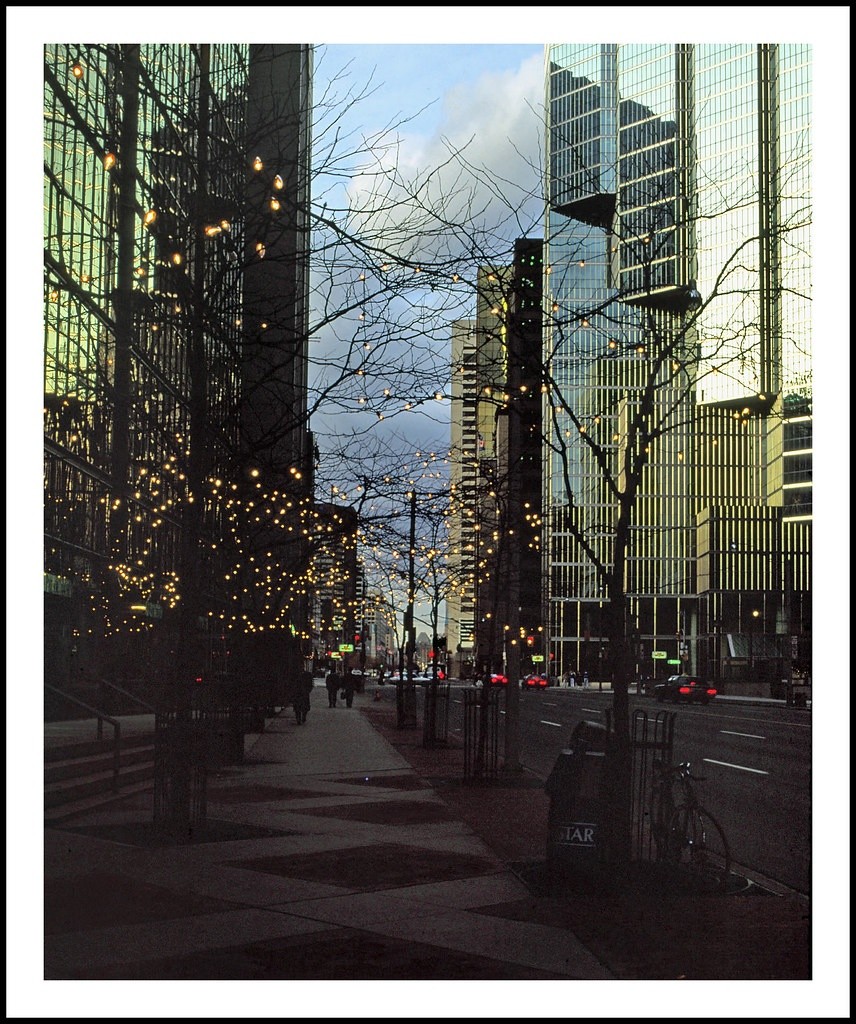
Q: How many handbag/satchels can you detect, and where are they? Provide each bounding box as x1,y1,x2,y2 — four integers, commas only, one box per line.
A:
340,692,345,699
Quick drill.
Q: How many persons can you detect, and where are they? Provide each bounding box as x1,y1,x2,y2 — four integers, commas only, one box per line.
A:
341,666,358,708
326,668,341,707
562,670,590,688
290,662,314,724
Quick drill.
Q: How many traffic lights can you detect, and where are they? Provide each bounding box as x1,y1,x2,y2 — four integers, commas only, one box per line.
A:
526,636,534,647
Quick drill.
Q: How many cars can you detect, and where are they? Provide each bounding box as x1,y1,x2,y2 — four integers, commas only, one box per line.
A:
374,666,444,686
351,670,370,677
482,674,508,686
521,673,547,691
654,675,717,704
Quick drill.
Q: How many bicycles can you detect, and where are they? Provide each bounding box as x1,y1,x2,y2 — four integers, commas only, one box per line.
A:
649,758,733,891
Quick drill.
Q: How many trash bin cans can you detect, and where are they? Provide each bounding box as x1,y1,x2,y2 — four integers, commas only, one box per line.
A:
543,719,614,880
795,692,808,707
